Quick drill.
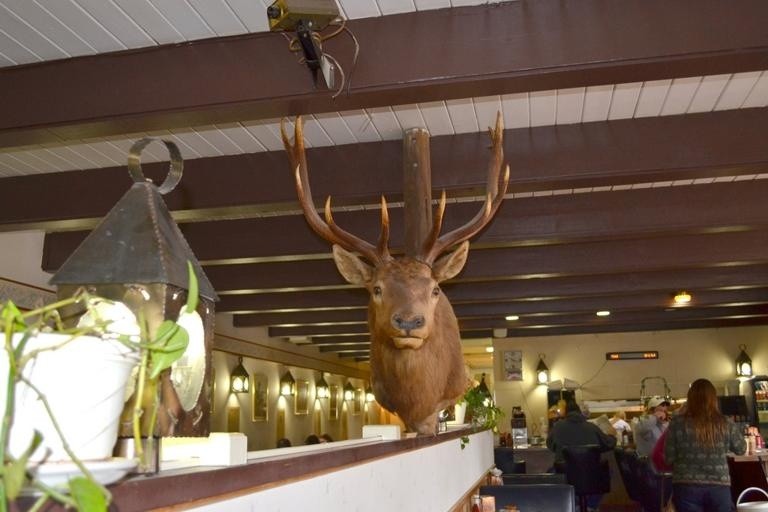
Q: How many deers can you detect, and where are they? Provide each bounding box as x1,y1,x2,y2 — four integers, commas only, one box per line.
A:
257,109,510,433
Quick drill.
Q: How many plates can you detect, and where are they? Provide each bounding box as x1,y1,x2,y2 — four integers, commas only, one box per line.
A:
23,457,136,497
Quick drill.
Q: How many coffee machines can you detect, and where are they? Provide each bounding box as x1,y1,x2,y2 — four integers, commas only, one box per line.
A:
511,406,528,451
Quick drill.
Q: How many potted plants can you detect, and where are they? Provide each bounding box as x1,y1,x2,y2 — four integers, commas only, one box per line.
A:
0,258,201,512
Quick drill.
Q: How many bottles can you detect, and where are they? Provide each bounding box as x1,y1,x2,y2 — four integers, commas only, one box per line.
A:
471,493,482,512
622,426,628,447
499,432,512,447
755,386,768,400
742,423,763,456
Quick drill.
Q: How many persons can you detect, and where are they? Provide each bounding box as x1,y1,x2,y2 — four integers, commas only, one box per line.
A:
609,408,631,448
631,395,675,478
319,433,333,442
277,438,292,447
664,378,747,512
545,399,618,511
303,434,320,445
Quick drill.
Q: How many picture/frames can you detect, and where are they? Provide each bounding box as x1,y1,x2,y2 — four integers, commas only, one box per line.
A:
250,375,361,424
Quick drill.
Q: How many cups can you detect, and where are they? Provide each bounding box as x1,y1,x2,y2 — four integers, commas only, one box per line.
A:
516,459,526,473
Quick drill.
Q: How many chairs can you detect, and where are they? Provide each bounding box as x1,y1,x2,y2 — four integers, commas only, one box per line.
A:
478,484,576,512
488,473,570,487
615,446,672,512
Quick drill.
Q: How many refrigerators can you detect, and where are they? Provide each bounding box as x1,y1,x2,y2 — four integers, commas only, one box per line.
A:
738,375,768,445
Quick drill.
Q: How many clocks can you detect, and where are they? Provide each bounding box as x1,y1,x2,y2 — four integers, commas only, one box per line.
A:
501,349,522,370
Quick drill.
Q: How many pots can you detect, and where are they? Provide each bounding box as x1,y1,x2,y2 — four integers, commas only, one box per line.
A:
1,330,141,465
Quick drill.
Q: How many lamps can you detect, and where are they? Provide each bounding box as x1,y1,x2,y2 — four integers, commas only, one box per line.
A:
50,134,220,446
673,290,695,306
227,354,251,395
315,369,329,398
735,343,754,378
278,370,296,397
364,387,375,402
535,353,550,384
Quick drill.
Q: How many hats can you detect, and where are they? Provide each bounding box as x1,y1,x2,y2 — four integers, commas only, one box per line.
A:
647,394,671,413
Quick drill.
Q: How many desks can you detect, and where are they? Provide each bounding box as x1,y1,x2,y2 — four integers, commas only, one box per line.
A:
724,447,767,483
